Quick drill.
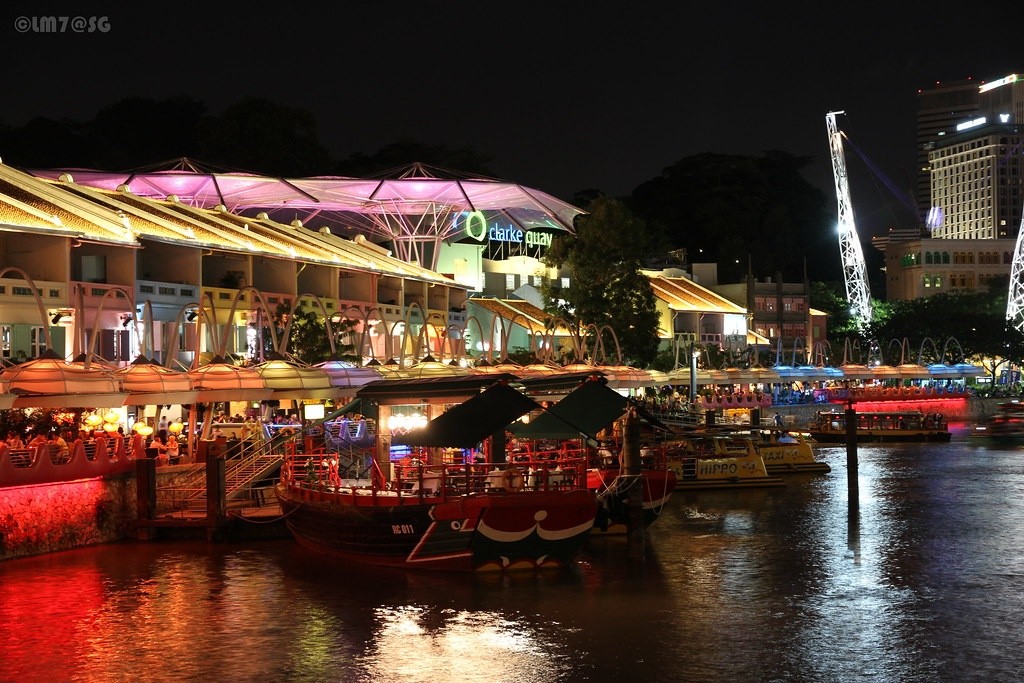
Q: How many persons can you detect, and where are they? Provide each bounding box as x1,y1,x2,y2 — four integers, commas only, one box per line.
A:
924,413,944,429
1,413,363,466
629,396,698,410
770,413,785,427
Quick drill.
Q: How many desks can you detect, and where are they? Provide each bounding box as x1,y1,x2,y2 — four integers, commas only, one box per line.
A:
485,465,513,491
546,465,572,491
414,469,445,497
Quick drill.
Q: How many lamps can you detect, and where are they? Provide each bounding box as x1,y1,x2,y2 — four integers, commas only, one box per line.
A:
49,311,63,324
120,316,134,327
185,311,198,322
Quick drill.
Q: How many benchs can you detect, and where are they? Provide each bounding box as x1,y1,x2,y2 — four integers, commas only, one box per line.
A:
284,452,340,488
8,447,37,468
83,442,98,461
48,442,75,464
145,448,162,466
106,438,130,457
166,448,182,466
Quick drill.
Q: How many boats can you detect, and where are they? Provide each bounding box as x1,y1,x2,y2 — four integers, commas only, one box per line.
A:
271,374,834,580
970,401,1024,444
800,404,957,443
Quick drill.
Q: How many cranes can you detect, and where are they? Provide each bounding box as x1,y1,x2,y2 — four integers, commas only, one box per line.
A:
825,110,875,350
